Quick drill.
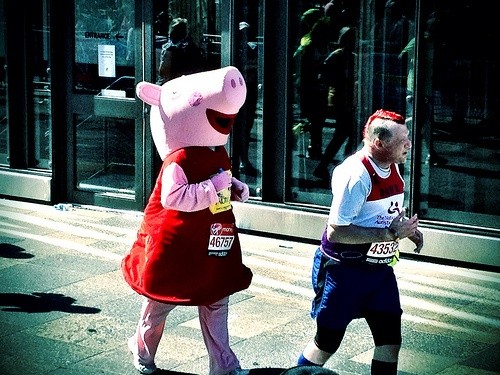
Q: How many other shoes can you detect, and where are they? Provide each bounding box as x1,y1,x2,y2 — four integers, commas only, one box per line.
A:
126,334,157,375
225,368,250,375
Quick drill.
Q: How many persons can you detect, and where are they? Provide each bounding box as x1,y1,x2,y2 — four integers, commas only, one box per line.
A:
120,66,253,375
296,109,423,375
127,0,449,184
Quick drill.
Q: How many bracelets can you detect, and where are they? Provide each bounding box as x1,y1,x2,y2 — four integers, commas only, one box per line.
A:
384,226,399,242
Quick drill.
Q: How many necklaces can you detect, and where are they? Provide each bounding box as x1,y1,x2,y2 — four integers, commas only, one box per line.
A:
368,156,390,173
